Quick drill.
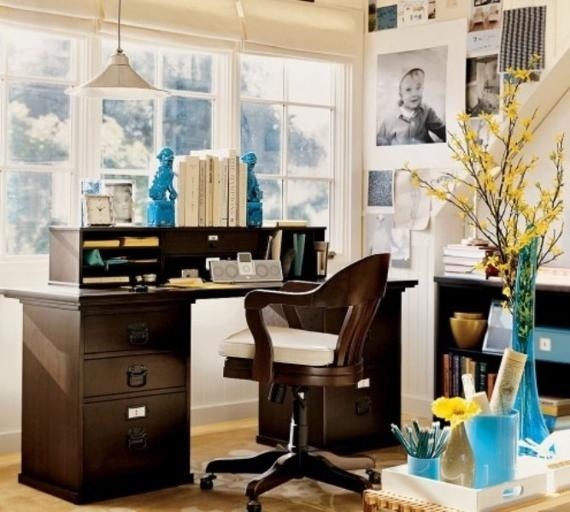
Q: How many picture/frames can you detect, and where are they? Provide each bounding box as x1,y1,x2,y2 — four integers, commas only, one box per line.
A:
106,179,133,223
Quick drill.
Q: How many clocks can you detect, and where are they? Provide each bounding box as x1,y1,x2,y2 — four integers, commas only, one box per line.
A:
84,194,116,228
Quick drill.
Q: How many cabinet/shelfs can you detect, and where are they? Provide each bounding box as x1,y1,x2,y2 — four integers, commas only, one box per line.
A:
431,274,569,407
48,222,330,286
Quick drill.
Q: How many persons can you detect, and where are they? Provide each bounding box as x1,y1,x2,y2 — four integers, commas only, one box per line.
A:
376,68,446,146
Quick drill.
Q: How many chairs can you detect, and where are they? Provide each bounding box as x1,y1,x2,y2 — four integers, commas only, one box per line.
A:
197,252,395,511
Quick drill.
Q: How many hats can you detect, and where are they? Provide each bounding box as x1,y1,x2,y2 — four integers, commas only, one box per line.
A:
398,54,425,84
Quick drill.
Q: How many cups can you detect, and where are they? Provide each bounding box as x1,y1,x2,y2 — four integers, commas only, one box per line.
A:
461,407,518,488
407,450,439,480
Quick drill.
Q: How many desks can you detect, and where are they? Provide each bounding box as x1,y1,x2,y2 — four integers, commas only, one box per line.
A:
2,271,418,505
362,434,570,511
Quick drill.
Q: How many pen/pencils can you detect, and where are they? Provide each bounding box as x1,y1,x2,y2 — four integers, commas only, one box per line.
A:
388,419,450,459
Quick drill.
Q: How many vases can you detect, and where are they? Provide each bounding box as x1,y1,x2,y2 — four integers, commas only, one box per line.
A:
406,455,440,482
439,421,477,489
402,51,569,437
498,228,556,457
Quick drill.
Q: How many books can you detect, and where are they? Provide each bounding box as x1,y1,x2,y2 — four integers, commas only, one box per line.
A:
83,234,160,284
294,232,307,277
270,228,284,260
168,276,203,286
441,353,497,402
171,148,248,228
441,243,508,283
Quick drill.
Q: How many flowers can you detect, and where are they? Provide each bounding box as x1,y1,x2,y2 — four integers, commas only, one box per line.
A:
429,395,483,427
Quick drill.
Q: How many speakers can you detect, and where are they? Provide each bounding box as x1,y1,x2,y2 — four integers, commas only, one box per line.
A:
209,252,284,283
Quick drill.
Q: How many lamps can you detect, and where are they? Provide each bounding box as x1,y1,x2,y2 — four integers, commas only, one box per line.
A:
64,1,172,102
